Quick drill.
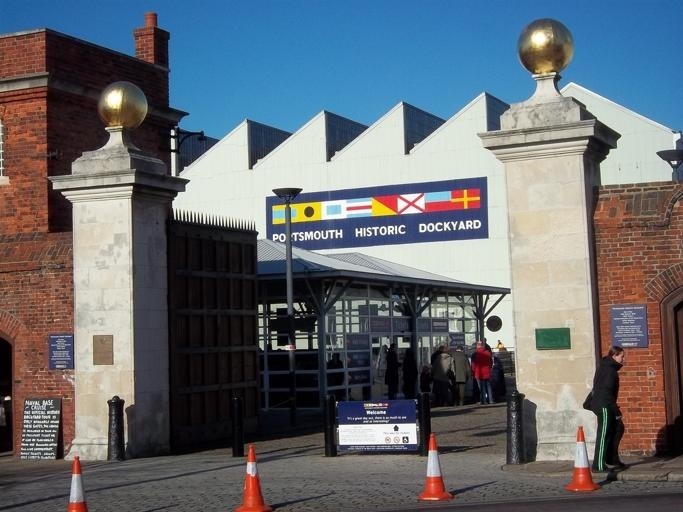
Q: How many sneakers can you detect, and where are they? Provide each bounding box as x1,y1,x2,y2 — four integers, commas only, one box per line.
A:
433,399,496,406
591,460,625,473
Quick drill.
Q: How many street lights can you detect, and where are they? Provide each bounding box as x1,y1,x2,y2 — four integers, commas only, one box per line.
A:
272,185,303,430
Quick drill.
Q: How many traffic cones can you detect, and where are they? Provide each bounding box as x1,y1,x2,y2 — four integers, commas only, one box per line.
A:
417,431,455,501
230,442,272,512
65,455,88,511
563,424,601,491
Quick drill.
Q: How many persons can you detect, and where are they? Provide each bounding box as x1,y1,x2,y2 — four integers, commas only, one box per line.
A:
589,345,630,475
375,336,497,407
325,351,345,390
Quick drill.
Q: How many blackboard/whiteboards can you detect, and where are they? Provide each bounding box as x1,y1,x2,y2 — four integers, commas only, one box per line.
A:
20,397,62,460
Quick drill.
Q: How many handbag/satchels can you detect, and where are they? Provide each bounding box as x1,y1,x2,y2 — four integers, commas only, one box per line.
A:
582,392,593,411
